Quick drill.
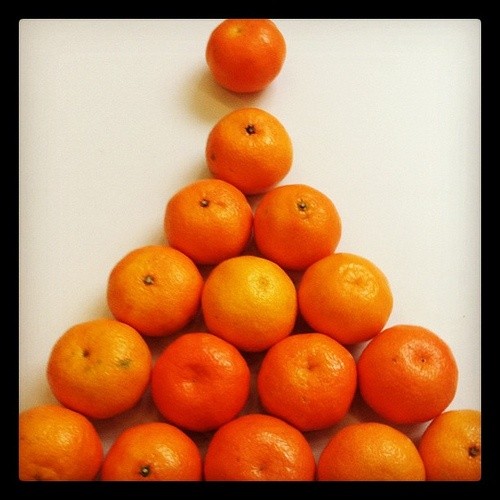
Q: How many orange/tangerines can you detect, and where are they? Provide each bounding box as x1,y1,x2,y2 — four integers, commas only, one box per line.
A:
205,19,287,92
19,107,481,481
